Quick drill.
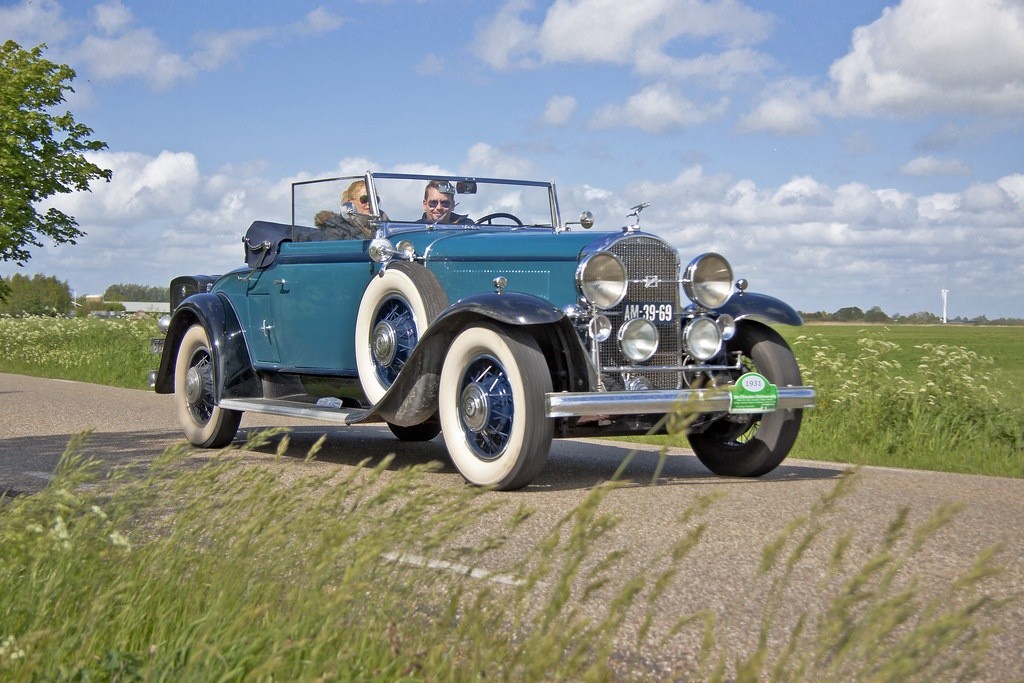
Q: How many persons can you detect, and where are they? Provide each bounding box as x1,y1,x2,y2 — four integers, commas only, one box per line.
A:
315,180,390,240
415,181,475,225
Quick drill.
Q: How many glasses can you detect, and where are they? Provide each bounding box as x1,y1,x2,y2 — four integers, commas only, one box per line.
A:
360,195,380,204
429,200,450,208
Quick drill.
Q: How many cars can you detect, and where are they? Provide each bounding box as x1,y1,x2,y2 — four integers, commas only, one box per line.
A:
146,169,817,493
